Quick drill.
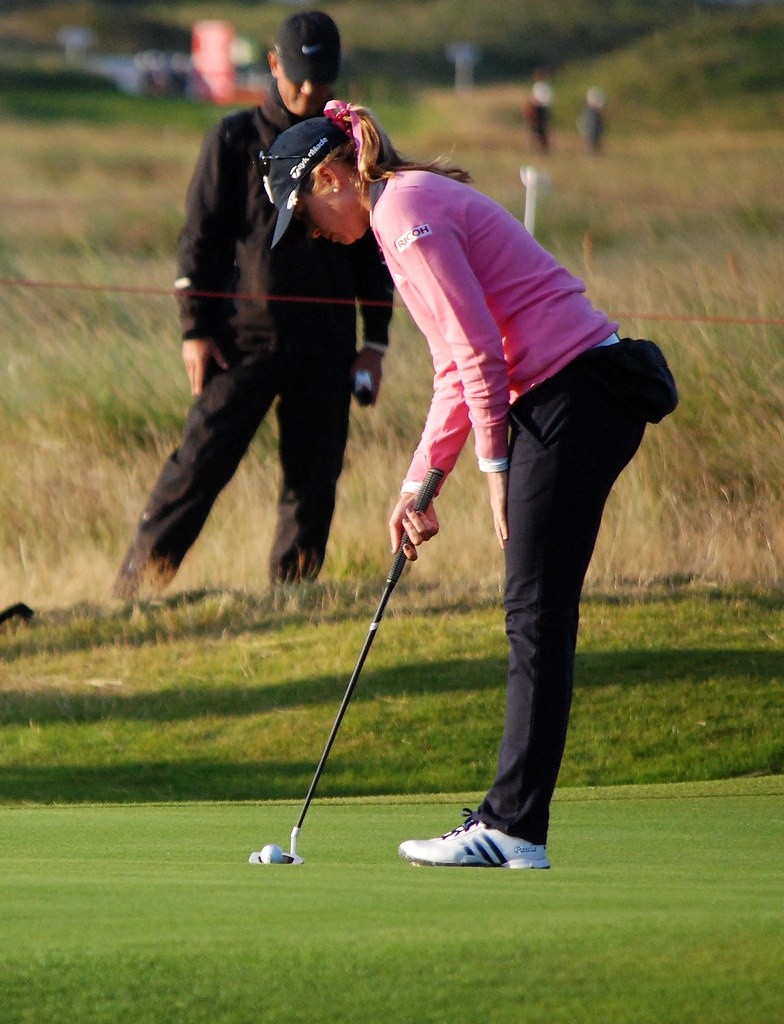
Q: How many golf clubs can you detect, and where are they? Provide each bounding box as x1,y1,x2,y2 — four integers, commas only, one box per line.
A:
248,466,446,867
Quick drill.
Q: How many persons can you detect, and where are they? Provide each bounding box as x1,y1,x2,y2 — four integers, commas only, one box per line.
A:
266,98,650,871
107,9,396,605
129,30,258,97
523,64,609,151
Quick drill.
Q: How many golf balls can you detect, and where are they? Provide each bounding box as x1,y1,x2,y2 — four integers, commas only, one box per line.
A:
259,842,283,866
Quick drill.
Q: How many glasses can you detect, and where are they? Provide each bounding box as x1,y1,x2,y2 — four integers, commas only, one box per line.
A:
258,150,322,176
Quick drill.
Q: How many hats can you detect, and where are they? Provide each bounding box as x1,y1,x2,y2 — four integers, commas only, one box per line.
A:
274,11,341,84
263,116,349,249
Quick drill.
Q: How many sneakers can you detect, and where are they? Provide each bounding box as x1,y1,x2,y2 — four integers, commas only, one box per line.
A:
398,808,551,869
